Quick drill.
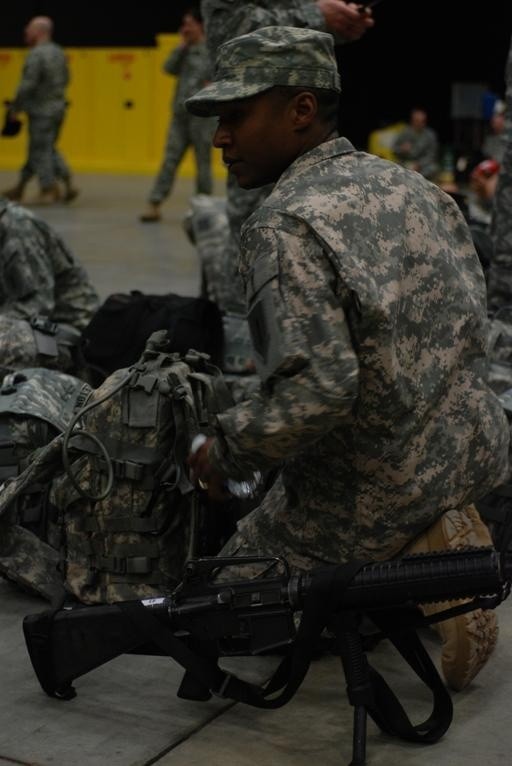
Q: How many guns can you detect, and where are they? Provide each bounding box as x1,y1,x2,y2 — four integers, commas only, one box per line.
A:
20,542,511,766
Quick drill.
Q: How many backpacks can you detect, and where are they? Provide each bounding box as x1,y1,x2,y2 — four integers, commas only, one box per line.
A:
0,288,229,608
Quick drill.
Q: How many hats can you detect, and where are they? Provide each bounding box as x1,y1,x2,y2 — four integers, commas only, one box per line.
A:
183,25,343,119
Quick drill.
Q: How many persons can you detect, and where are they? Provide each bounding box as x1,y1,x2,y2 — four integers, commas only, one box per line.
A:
480,92,501,168
0,193,105,377
0,14,70,205
485,47,512,324
189,20,501,687
37,156,85,203
389,104,444,185
202,0,386,318
138,12,215,222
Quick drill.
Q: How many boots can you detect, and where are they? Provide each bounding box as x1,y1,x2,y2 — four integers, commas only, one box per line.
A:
405,502,499,694
139,201,161,223
0,181,79,204
